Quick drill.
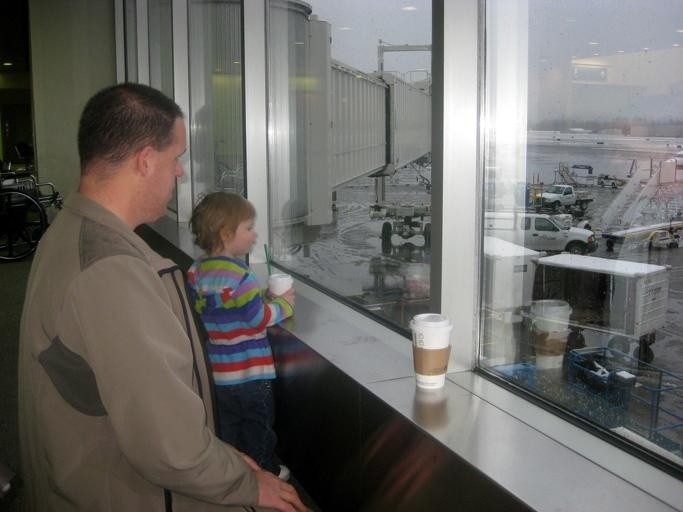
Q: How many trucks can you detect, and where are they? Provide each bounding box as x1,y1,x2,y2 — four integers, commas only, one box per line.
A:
482,171,679,259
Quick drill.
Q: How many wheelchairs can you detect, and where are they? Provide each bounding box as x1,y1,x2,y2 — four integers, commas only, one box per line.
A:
0,158,67,264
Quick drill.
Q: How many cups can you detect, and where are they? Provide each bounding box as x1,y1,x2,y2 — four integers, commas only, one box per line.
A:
527,297,571,373
406,313,454,389
411,386,452,432
269,275,293,300
399,260,429,298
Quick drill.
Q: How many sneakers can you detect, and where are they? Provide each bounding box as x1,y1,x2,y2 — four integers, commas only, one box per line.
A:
277,464,292,482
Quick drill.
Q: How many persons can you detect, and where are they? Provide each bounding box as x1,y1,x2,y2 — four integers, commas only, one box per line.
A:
15,81,311,511
184,189,297,482
